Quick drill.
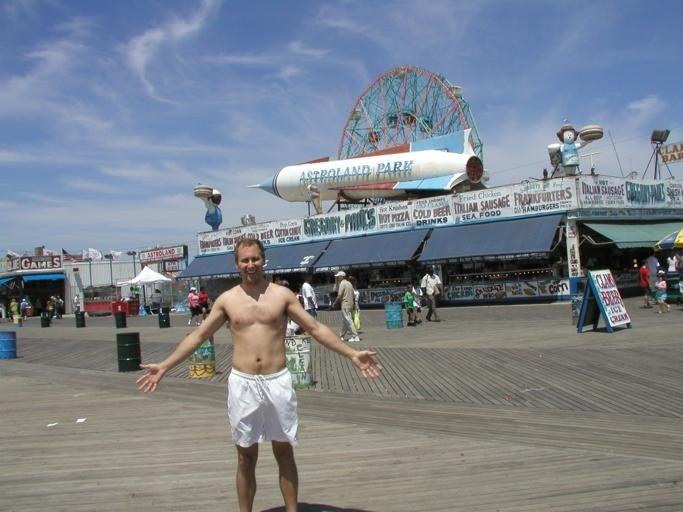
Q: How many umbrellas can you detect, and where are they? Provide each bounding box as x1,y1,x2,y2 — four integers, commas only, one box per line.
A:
116,265,172,308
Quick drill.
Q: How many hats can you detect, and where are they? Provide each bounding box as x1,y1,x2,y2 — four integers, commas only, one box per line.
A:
334,271,345,277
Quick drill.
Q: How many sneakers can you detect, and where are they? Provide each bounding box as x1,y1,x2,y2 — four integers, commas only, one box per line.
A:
340,336,344,341
348,337,359,341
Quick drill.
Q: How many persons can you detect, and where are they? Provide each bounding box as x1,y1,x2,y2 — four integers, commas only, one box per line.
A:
302,272,318,317
639,261,653,308
412,280,423,322
10,299,19,315
136,239,383,512
403,285,417,326
149,289,162,309
654,272,671,314
21,299,29,316
47,296,56,324
197,289,209,313
347,276,361,333
332,271,361,342
55,296,64,318
647,252,659,281
420,266,443,321
188,288,199,324
667,251,676,271
73,294,80,314
